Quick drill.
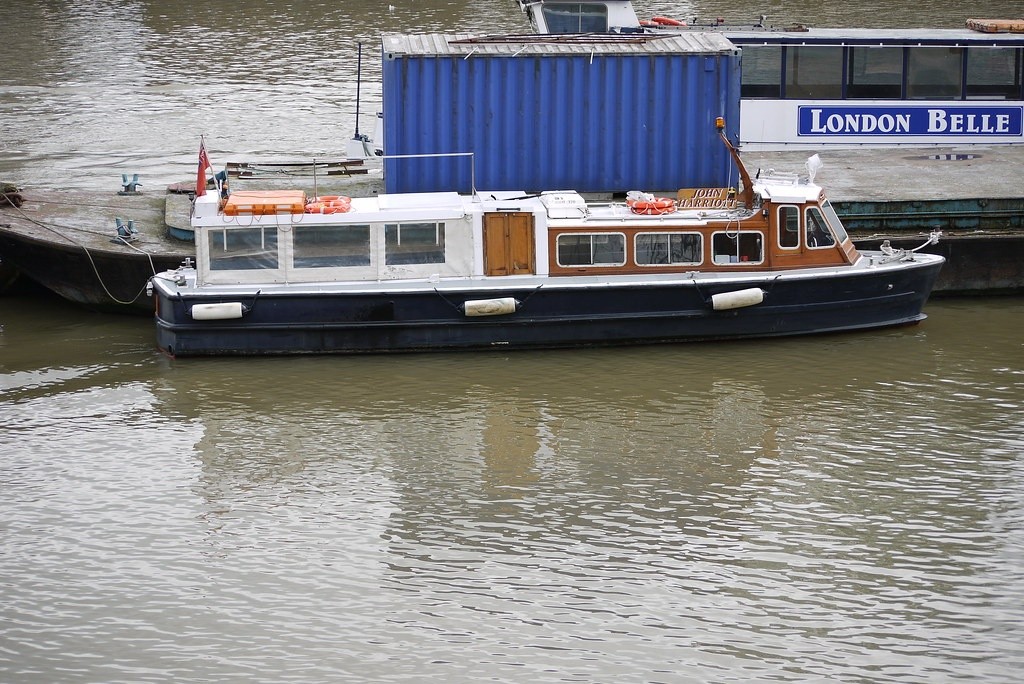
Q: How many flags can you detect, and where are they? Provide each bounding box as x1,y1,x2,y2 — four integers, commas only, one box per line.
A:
197,136,212,197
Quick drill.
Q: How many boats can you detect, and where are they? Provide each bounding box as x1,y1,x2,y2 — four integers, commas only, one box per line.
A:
517,0,1024,151
146,133,946,358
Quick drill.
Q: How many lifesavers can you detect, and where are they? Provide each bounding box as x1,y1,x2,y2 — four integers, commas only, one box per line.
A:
639,19,659,26
303,202,351,213
625,196,676,209
307,194,352,205
652,16,687,27
630,207,678,215
